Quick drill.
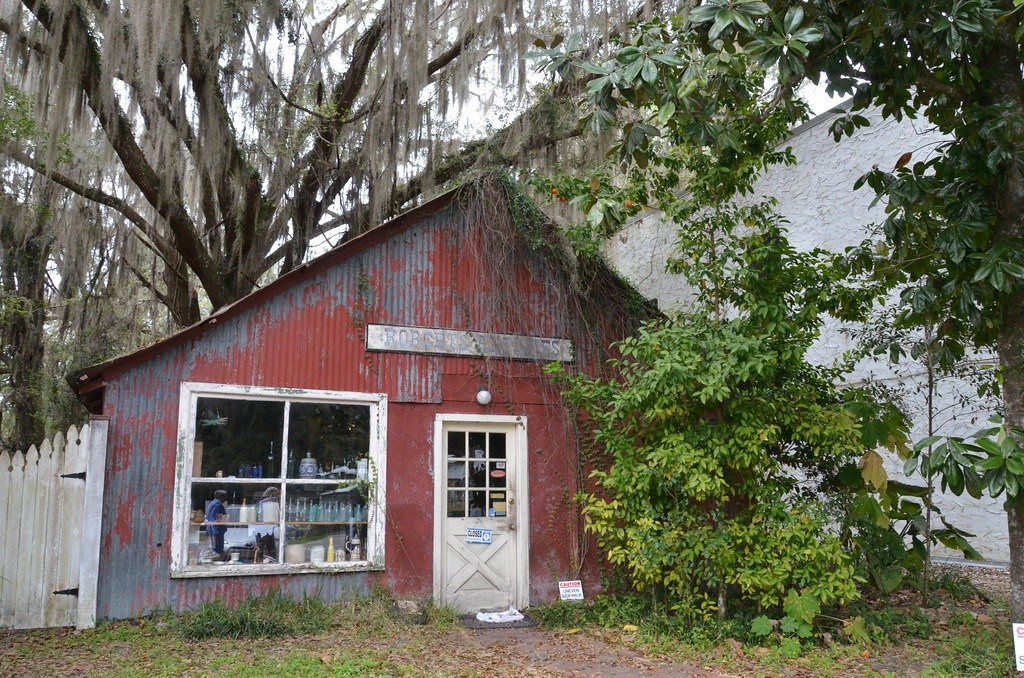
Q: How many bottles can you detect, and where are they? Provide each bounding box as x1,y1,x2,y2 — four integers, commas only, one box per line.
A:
239,499,256,522
239,463,264,478
266,440,278,478
286,497,368,522
288,451,297,478
327,537,334,562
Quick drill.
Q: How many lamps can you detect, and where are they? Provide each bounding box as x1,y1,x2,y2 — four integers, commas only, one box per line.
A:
477,385,491,404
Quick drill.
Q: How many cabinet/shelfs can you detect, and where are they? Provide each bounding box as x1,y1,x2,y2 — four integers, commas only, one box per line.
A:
187,477,369,564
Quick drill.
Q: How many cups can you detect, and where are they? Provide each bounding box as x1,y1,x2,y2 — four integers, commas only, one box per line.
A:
216,470,223,477
310,545,325,562
231,553,240,561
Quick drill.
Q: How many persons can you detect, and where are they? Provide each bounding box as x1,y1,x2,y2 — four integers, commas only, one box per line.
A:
256,487,280,564
207,490,227,554
